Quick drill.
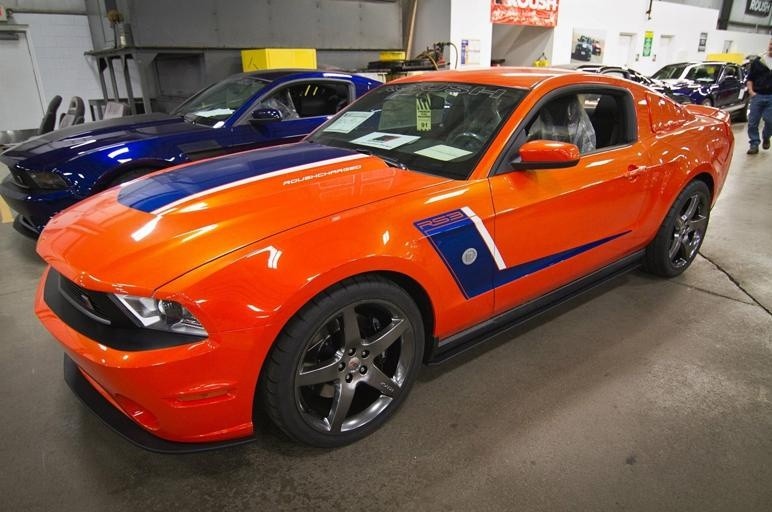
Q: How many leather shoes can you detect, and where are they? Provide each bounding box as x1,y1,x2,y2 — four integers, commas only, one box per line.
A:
763,140,770,149
748,147,758,153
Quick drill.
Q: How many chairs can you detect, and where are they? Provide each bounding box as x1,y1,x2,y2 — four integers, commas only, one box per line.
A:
422,92,503,151
590,93,624,150
37,95,62,134
527,95,596,153
59,96,84,129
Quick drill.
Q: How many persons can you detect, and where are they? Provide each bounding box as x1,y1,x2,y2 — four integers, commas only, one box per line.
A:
743,39,772,155
